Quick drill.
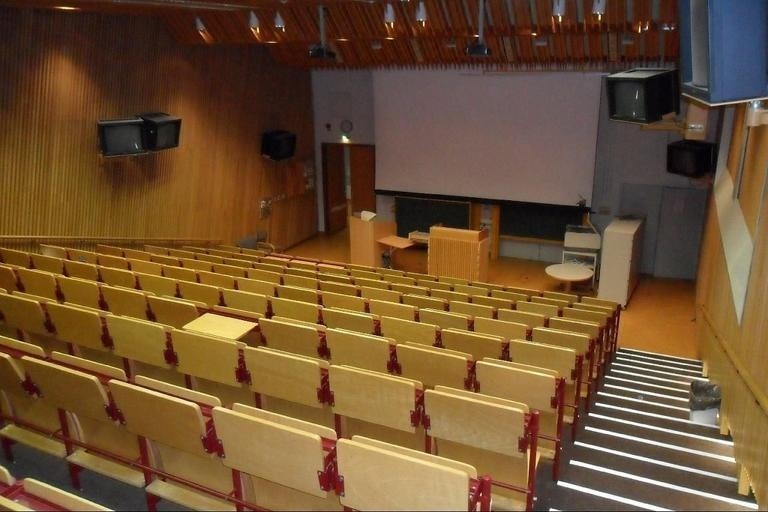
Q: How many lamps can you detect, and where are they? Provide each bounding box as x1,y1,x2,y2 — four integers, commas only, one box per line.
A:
187,1,610,61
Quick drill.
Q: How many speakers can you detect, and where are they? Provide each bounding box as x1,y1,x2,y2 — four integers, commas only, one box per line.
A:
262,130,296,161
679,0,768,104
666,140,717,178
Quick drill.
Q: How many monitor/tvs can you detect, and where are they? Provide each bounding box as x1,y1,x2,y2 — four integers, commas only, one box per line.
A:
142,112,182,151
97,116,147,157
605,67,680,124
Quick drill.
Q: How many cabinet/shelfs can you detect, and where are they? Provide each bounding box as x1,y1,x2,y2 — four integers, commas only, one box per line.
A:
561,223,601,290
350,212,397,268
596,217,642,308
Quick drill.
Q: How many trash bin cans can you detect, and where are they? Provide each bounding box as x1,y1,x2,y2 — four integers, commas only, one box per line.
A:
690,380,722,425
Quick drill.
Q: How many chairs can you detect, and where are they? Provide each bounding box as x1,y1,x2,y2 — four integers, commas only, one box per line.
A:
0,243,623,512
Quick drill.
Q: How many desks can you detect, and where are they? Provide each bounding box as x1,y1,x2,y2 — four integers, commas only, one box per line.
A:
427,223,489,283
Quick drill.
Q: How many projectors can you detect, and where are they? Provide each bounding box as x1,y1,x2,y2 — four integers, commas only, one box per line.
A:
467,45,491,56
309,49,336,59
